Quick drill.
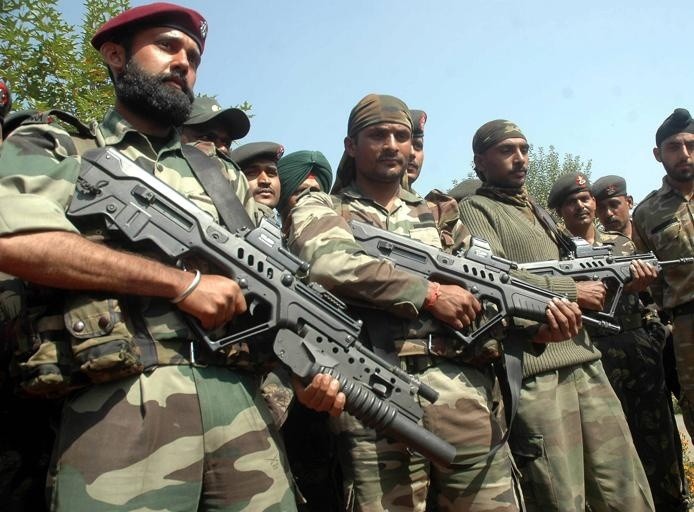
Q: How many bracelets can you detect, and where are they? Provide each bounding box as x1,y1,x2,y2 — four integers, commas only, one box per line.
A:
426,281,440,310
172,268,201,302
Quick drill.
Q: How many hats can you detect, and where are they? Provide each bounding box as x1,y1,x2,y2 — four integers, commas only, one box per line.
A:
0,81,12,120
655,107,694,144
91,3,207,54
548,172,589,208
591,175,627,198
448,179,483,200
183,96,250,141
4,110,42,138
231,142,285,166
407,109,429,136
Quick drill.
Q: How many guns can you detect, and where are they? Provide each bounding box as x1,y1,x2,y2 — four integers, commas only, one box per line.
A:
510,236,694,320
346,218,622,346
72,138,459,466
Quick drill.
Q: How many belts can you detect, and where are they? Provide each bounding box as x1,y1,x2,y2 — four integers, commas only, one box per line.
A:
586,313,645,335
155,338,222,366
395,334,468,361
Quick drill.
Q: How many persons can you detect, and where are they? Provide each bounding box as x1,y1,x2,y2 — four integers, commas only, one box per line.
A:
456,119,654,512
407,109,427,199
0,1,344,512
230,141,285,207
548,171,689,512
180,97,251,157
275,150,333,227
289,91,583,512
632,109,694,448
592,175,694,499
